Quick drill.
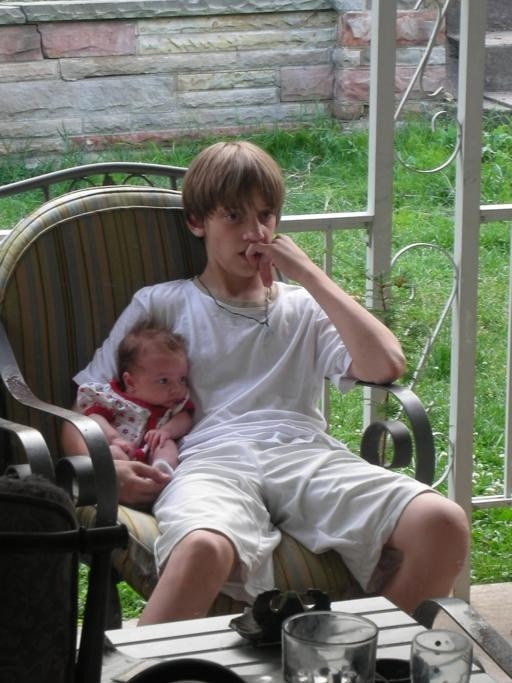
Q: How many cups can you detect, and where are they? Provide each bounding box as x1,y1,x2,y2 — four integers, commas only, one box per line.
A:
409,629,473,683
281,611,378,683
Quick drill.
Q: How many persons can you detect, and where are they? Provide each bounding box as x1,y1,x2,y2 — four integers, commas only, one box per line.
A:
57,136,472,630
79,312,196,515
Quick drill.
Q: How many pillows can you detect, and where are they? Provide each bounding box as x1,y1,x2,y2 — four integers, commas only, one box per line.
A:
1,186,207,465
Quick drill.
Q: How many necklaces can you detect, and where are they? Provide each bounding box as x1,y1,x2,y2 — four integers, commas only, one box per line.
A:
193,272,272,329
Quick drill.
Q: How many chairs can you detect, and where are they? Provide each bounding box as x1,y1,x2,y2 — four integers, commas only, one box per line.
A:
1,418,129,683
1,162,435,628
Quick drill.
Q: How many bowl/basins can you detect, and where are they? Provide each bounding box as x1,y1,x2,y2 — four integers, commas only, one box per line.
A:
374,658,429,683
